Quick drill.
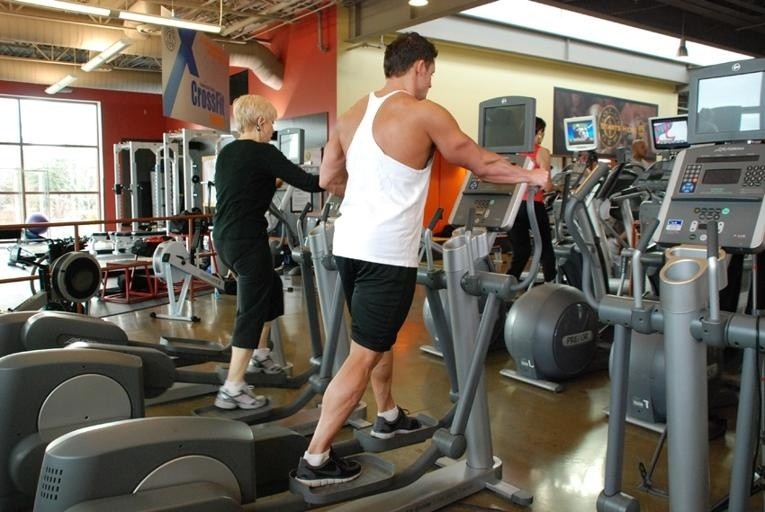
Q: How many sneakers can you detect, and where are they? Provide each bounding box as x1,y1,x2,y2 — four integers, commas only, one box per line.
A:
294,442,364,488
370,403,423,441
245,352,284,375
213,381,271,411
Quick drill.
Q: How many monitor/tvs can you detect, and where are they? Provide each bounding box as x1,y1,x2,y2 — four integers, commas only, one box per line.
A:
478,96,536,153
564,116,599,151
687,56,765,144
648,115,692,153
278,128,304,164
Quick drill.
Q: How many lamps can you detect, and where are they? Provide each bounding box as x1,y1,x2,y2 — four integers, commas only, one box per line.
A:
79,40,131,73
43,73,79,95
17,0,226,35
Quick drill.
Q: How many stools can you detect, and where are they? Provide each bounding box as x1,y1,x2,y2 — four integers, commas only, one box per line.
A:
100,258,153,306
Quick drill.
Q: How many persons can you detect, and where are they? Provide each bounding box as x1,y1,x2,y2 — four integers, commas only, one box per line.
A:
294,31,552,488
212,95,331,412
630,139,655,171
504,116,564,285
575,150,598,172
